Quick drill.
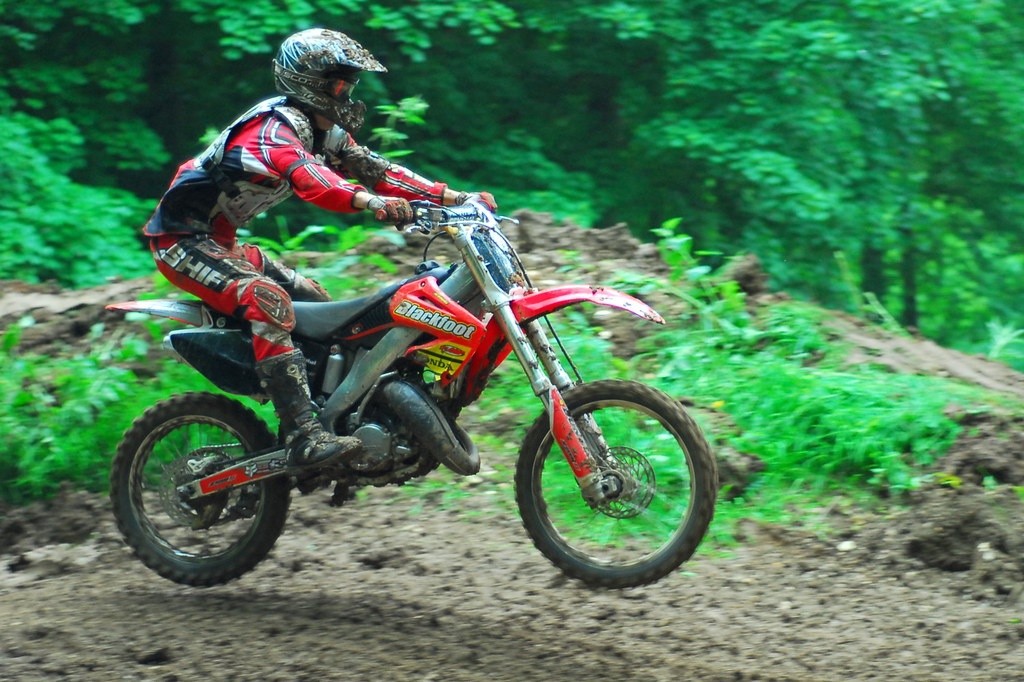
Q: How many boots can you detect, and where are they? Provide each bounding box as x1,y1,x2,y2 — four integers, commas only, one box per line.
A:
259,348,361,473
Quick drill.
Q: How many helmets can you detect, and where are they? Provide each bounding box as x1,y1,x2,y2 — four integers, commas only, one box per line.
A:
270,27,388,135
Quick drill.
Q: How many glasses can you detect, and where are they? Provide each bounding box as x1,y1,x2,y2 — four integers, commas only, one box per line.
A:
328,77,361,99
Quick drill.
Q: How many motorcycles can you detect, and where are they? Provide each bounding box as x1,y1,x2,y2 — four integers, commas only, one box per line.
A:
110,195,719,590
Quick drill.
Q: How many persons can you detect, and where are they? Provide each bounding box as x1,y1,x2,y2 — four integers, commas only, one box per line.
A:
142,28,498,478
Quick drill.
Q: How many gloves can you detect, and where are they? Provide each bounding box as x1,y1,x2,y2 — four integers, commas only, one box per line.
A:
454,190,499,212
369,196,414,233
